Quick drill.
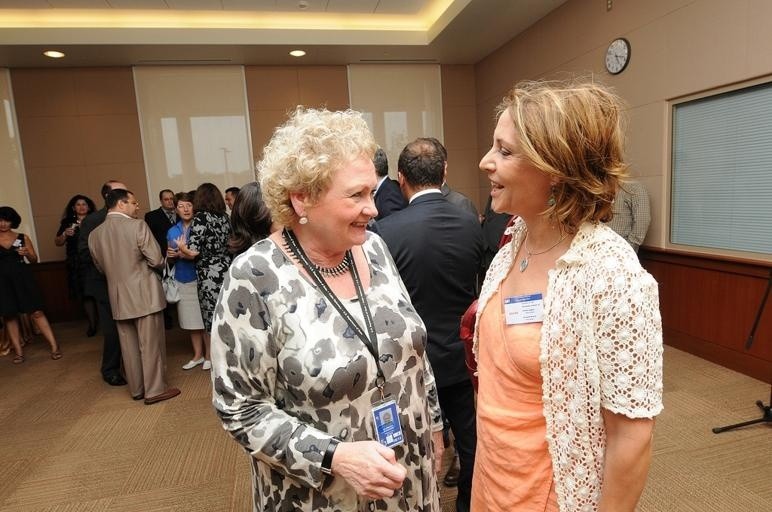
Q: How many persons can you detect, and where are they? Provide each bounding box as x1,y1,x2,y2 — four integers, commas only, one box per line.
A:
466,69,666,511
602,181,651,253
54,179,282,405
0,205,62,364
209,104,446,511
373,135,511,510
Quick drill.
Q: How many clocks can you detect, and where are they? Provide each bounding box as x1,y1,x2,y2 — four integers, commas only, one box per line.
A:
604,38,631,75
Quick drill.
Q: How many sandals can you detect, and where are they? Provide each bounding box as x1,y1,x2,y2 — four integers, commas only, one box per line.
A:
51,345,63,360
13,354,24,364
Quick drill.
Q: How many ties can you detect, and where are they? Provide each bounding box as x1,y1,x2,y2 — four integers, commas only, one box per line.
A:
166,212,175,224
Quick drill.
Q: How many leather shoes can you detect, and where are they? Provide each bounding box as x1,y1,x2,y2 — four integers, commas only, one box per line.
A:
182,357,205,370
133,389,181,405
443,456,461,487
202,360,211,370
104,375,127,386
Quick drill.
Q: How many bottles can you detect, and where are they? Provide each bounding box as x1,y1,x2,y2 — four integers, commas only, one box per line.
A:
17,244,24,263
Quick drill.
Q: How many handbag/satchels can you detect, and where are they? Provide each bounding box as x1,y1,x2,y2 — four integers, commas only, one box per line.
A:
460,299,478,395
162,256,181,303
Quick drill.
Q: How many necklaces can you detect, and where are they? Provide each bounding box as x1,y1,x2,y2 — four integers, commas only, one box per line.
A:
518,230,569,273
282,235,350,278
